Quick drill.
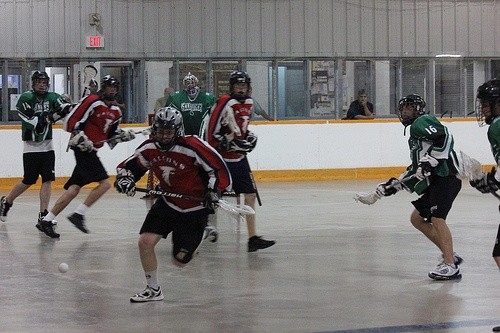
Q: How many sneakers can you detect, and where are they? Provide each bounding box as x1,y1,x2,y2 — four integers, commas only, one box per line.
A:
247,237,275,251
436,252,463,269
130,285,163,302
37,212,58,225
0,195,12,222
428,264,461,280
68,213,88,234
36,220,60,238
205,223,218,242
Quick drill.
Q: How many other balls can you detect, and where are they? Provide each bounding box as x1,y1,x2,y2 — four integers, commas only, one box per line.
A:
59,263,69,273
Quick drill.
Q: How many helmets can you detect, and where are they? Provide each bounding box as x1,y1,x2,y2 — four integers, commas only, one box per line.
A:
100,74,119,100
183,72,201,101
230,71,251,102
475,79,500,127
152,107,183,149
396,94,425,126
32,70,49,94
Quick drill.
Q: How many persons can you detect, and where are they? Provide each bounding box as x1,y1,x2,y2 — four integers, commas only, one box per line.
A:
89,78,99,94
35,76,135,238
377,93,463,280
346,89,374,119
470,76,500,271
246,86,279,121
155,87,176,113
117,106,218,301
0,71,87,224
164,73,218,136
199,70,276,252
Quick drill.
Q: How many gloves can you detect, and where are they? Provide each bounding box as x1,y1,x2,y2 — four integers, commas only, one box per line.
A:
114,129,135,143
69,130,97,153
46,108,67,123
200,189,219,211
415,153,439,181
114,175,136,197
375,176,401,197
247,130,257,149
469,166,500,193
227,138,253,153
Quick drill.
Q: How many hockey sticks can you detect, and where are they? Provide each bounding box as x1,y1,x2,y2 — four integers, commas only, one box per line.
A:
66,65,97,152
355,172,432,205
458,148,500,200
134,187,256,216
94,127,153,145
221,106,262,206
